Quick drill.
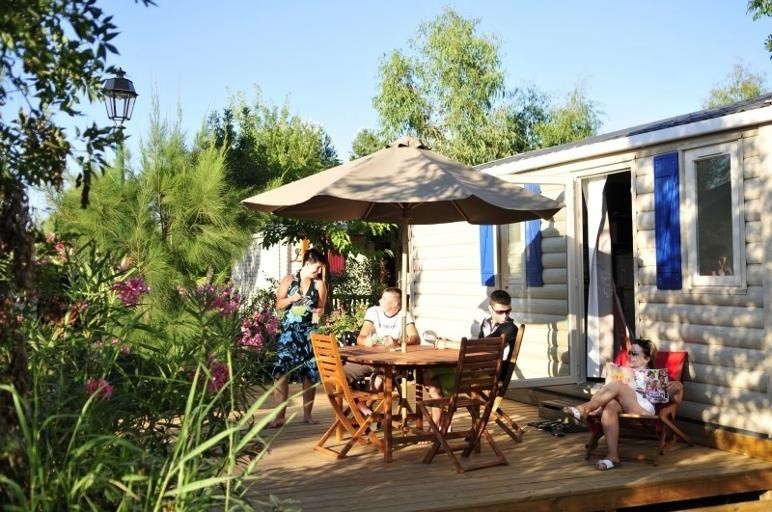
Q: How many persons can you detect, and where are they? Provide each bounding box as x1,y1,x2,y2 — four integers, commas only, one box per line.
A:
423,289,518,433
269,248,326,429
343,287,420,417
563,339,684,472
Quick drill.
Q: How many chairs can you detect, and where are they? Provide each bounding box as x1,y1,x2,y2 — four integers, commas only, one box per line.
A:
481,323,525,443
584,349,695,467
418,334,509,473
310,331,385,459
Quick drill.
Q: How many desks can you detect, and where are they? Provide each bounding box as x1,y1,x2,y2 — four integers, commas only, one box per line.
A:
339,345,481,462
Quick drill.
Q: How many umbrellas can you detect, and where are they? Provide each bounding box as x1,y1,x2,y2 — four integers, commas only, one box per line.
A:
240,139,564,419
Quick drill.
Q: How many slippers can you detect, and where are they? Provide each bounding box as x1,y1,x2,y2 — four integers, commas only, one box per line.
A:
595,459,621,471
563,407,589,426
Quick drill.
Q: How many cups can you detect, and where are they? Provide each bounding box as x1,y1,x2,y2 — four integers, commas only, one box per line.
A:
312,308,319,324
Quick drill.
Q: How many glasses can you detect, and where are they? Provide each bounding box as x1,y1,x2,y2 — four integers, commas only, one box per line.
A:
628,351,639,356
492,307,512,314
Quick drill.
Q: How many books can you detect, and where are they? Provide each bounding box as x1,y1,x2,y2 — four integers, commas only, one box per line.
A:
605,362,669,404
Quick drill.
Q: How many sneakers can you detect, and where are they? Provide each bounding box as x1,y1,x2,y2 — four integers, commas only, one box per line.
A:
370,422,381,431
